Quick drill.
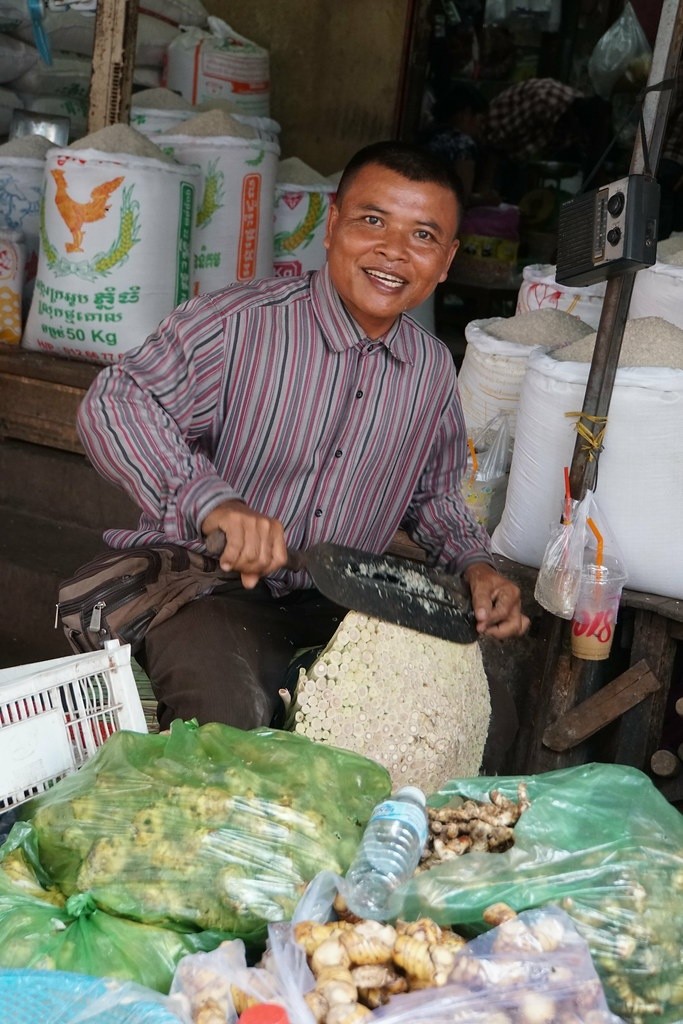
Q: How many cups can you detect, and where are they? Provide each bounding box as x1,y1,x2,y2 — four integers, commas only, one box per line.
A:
570,553,629,661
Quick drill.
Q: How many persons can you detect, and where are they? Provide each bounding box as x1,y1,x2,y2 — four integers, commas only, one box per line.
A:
411,75,610,212
74,136,530,777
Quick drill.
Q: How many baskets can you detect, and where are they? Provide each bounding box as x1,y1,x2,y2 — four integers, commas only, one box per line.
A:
0,637,149,815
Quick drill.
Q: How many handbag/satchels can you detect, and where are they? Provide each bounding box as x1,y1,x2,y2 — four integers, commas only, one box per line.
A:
55,544,228,655
0,713,683,1024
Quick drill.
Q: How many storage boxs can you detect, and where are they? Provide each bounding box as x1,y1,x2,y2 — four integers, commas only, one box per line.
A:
0,640,152,817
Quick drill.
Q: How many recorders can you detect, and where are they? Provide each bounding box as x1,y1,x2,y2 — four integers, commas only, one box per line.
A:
556,174,661,289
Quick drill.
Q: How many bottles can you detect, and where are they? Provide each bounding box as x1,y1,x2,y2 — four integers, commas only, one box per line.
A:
342,786,429,920
